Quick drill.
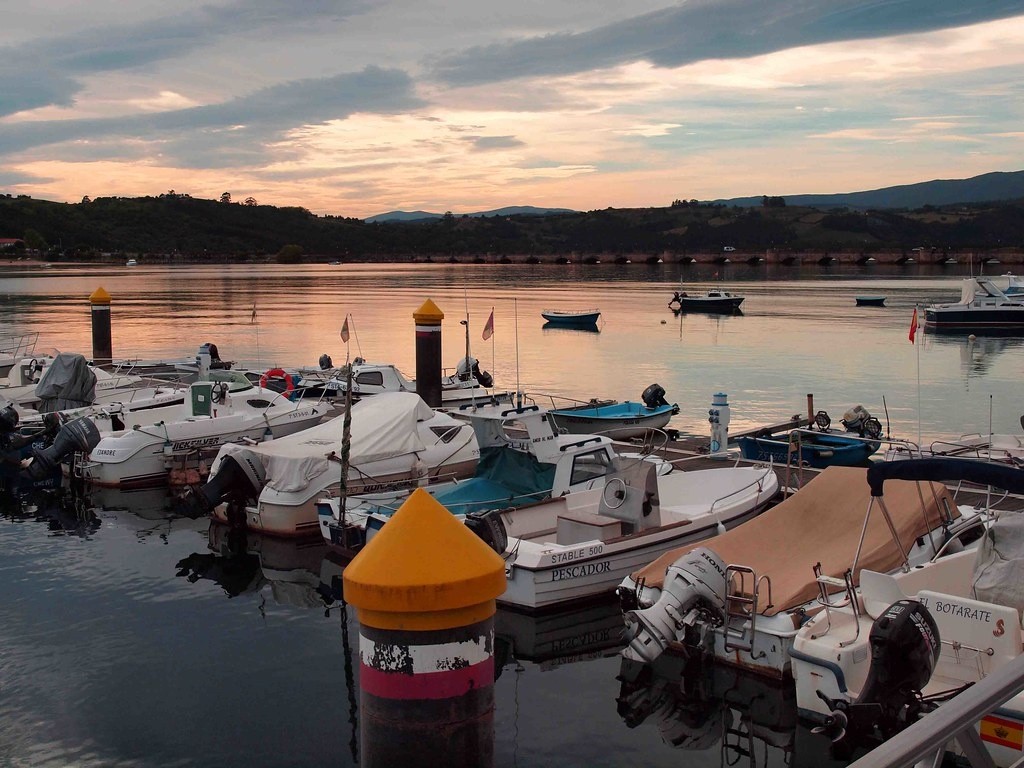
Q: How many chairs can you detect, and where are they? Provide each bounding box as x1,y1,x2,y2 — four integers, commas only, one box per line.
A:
858,569,918,621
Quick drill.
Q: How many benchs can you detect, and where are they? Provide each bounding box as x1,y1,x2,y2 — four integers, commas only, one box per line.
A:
556,511,621,546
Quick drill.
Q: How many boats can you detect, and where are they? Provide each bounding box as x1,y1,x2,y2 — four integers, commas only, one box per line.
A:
856,296,887,305
524,383,680,440
0,330,40,378
787,508,1024,768
312,297,672,560
458,464,782,609
738,405,883,467
672,291,745,309
1,316,482,536
613,464,999,680
126,259,137,265
541,308,602,324
916,253,1024,335
884,395,1024,495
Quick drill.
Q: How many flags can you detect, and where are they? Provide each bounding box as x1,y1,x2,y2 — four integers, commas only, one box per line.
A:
340,318,349,343
482,311,493,340
909,308,917,344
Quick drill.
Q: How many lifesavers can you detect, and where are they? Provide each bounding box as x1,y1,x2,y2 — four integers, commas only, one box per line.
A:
260,368,294,399
